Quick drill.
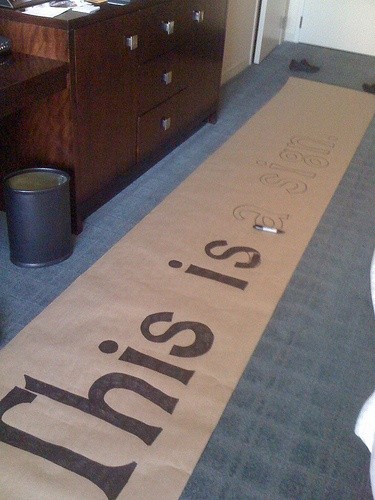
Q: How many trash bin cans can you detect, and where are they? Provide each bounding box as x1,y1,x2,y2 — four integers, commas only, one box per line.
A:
1,164,74,269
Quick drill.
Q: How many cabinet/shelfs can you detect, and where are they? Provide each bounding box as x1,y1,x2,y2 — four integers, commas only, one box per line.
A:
0,0,229,234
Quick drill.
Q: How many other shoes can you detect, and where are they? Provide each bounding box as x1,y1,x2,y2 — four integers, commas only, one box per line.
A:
290,58,320,72
363,83,375,94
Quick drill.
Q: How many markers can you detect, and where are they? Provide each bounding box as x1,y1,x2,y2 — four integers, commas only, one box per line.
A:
253,225,285,234
